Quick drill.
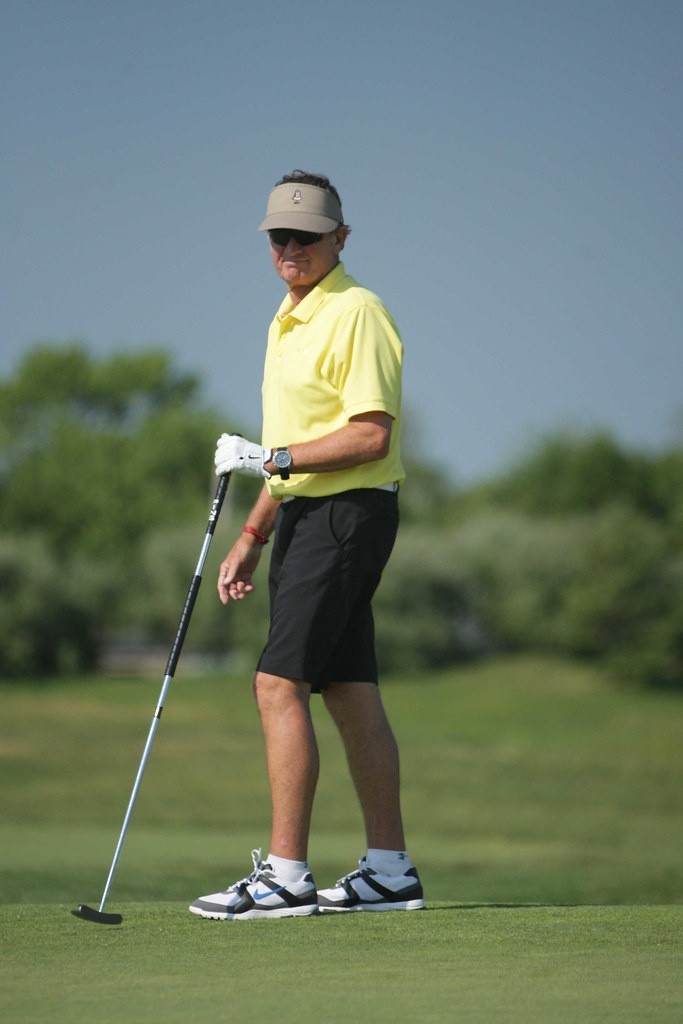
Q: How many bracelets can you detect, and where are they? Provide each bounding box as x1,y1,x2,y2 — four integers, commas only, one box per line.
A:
242,525,269,544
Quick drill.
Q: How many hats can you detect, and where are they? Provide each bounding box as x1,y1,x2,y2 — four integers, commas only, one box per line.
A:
255,182,346,233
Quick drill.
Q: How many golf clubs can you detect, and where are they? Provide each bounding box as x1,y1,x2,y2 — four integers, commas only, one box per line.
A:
70,432,244,925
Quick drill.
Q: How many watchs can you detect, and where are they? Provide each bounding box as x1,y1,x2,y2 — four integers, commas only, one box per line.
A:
272,446,291,480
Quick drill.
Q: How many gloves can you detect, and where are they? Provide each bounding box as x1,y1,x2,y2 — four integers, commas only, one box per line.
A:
214,433,274,480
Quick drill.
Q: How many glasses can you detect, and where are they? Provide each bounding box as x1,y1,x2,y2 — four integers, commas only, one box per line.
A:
267,231,323,246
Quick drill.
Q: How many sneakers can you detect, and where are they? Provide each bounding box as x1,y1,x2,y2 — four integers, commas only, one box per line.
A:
316,859,426,914
189,849,319,920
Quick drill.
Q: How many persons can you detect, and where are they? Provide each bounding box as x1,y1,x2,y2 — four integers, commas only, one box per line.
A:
186,168,426,920
